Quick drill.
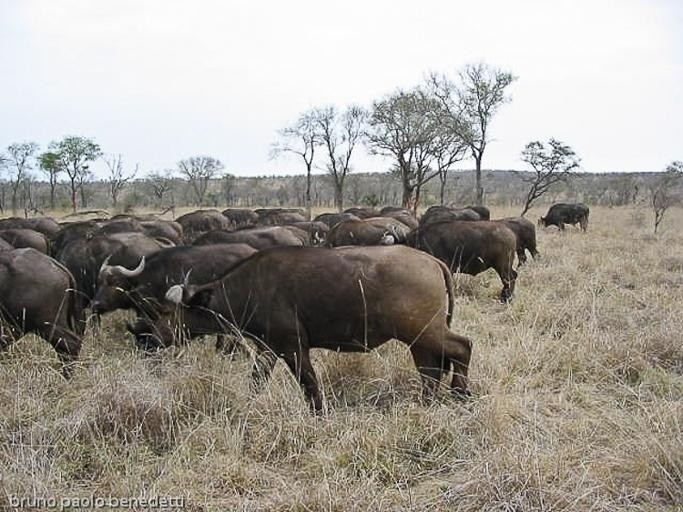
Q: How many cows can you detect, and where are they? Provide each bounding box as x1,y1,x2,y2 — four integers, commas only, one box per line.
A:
151,244,472,416
0,248,82,380
0,206,539,310
86,244,259,360
538,203,589,232
